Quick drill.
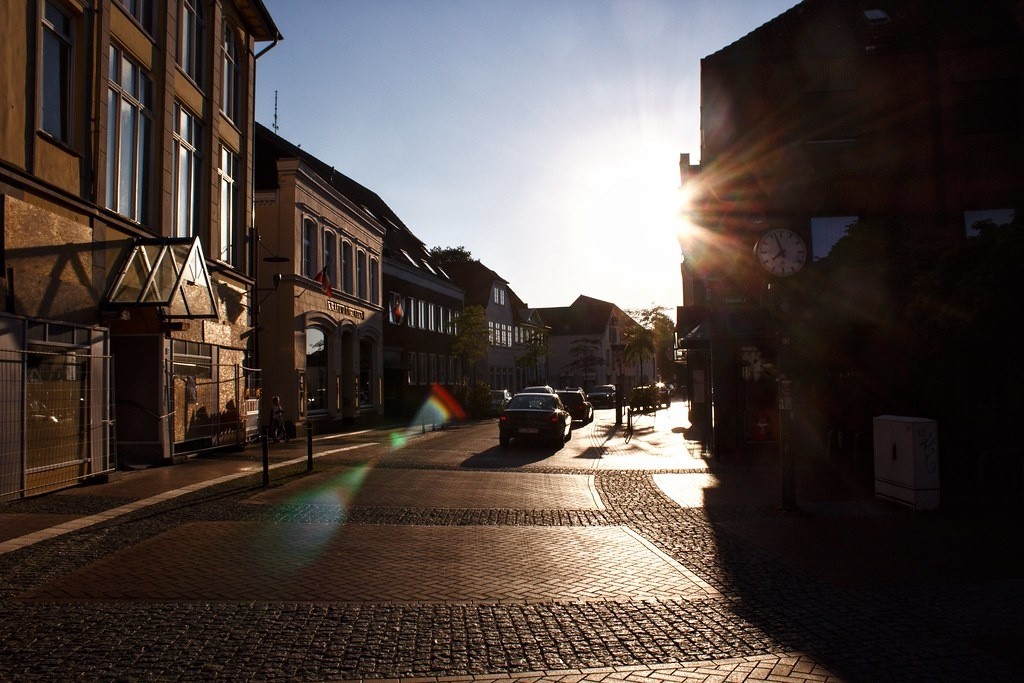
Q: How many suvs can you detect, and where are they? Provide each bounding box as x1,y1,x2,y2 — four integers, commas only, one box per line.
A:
586,384,618,408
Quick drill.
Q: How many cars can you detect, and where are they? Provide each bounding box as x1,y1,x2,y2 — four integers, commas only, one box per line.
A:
498,392,572,446
555,387,594,424
522,386,554,395
490,389,511,415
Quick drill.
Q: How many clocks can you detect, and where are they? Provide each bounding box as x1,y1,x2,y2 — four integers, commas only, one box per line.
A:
752,227,808,281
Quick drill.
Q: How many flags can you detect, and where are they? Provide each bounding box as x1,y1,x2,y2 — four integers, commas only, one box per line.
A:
395,302,403,325
314,270,332,299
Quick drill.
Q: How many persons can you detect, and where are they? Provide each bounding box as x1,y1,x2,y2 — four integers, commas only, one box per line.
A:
270,395,290,444
